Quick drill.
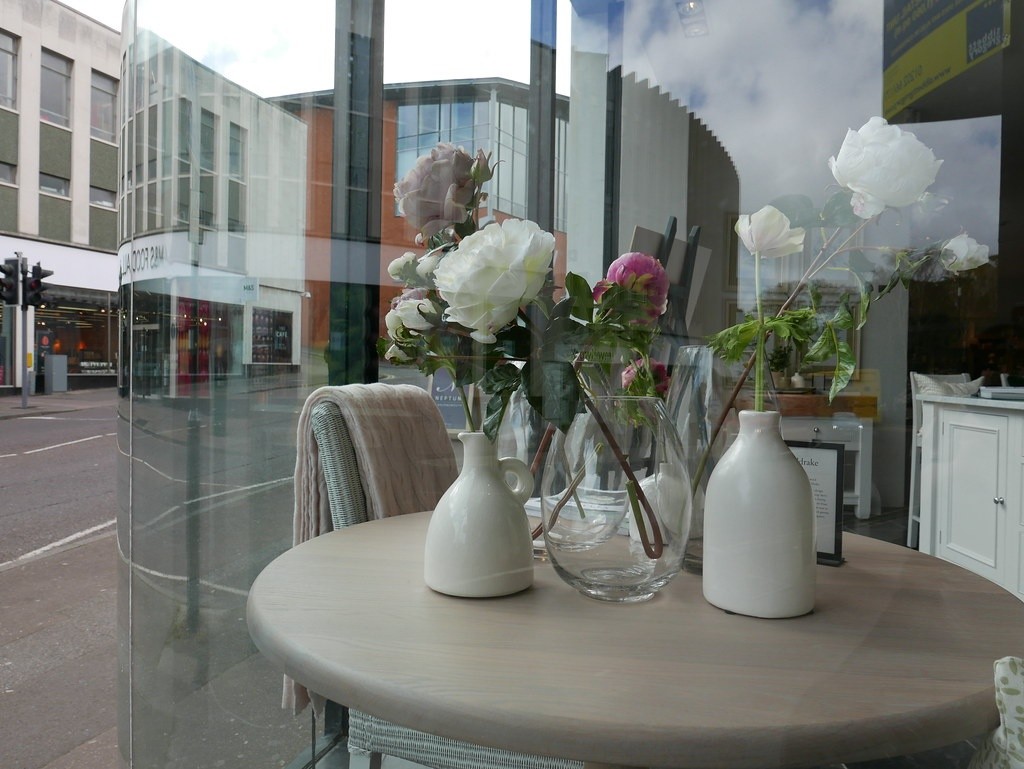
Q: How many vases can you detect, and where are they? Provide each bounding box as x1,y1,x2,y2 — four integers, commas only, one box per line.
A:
667,339,783,576
423,432,532,597
702,409,818,618
540,395,693,603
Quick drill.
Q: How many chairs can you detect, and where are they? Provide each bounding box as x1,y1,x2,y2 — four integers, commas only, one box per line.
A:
292,384,614,768
906,371,972,548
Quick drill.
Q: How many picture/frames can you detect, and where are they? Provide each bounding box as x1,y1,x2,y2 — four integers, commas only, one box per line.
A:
798,218,865,293
788,294,862,381
720,294,788,389
723,212,789,293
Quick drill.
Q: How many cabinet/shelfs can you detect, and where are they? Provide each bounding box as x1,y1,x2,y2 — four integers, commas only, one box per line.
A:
735,394,877,521
915,394,1024,605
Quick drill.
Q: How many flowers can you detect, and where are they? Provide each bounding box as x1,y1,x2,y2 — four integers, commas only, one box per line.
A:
383,142,593,445
570,250,672,394
705,115,990,410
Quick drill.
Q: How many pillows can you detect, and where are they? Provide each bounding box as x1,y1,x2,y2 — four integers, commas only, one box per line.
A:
914,373,984,438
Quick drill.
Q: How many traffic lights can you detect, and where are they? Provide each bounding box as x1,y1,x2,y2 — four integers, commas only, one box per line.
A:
22,277,41,308
33,261,54,306
0,257,19,307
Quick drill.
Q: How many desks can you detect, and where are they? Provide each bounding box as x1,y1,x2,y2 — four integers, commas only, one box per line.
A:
246,503,1024,769
